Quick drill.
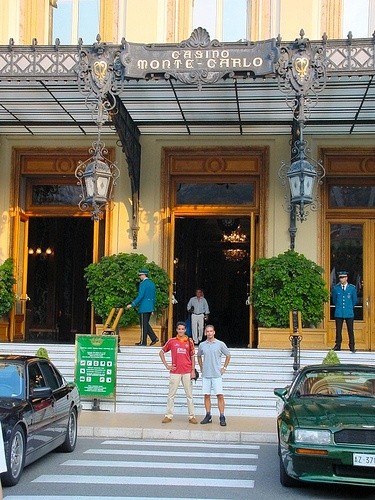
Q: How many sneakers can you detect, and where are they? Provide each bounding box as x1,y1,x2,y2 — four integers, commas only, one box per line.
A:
200,412,212,424
219,415,226,426
189,418,198,424
162,416,172,423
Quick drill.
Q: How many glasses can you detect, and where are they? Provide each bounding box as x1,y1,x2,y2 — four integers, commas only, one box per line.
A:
339,277,344,278
138,275,141,276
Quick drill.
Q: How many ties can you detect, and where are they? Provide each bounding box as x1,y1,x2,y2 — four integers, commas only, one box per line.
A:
343,285,345,291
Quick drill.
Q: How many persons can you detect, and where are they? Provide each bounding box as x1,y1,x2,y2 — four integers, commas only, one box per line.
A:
332,272,358,351
187,289,210,345
159,322,198,424
127,271,159,346
197,325,230,426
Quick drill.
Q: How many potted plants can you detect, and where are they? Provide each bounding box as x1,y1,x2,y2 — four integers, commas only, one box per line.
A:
252,250,328,348
0,258,16,342
84,252,169,346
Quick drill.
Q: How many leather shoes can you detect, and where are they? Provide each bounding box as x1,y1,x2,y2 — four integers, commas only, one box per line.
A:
135,342,147,346
332,344,340,351
150,337,159,346
349,346,354,351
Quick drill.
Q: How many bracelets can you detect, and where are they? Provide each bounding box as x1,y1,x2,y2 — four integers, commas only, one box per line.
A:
223,367,226,369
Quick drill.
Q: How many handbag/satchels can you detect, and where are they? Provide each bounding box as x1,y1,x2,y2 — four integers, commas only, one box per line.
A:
188,306,194,312
191,369,199,384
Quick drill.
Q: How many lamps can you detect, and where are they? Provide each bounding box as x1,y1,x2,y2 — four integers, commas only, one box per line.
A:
278,78,326,222
75,78,121,220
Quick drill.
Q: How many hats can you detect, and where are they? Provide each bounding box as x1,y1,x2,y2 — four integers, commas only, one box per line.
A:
138,269,149,275
337,271,349,277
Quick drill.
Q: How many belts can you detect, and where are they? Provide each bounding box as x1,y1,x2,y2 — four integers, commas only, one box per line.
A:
192,312,202,315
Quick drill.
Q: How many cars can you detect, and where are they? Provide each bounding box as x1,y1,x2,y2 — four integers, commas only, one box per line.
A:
274,364,375,489
0,355,80,488
